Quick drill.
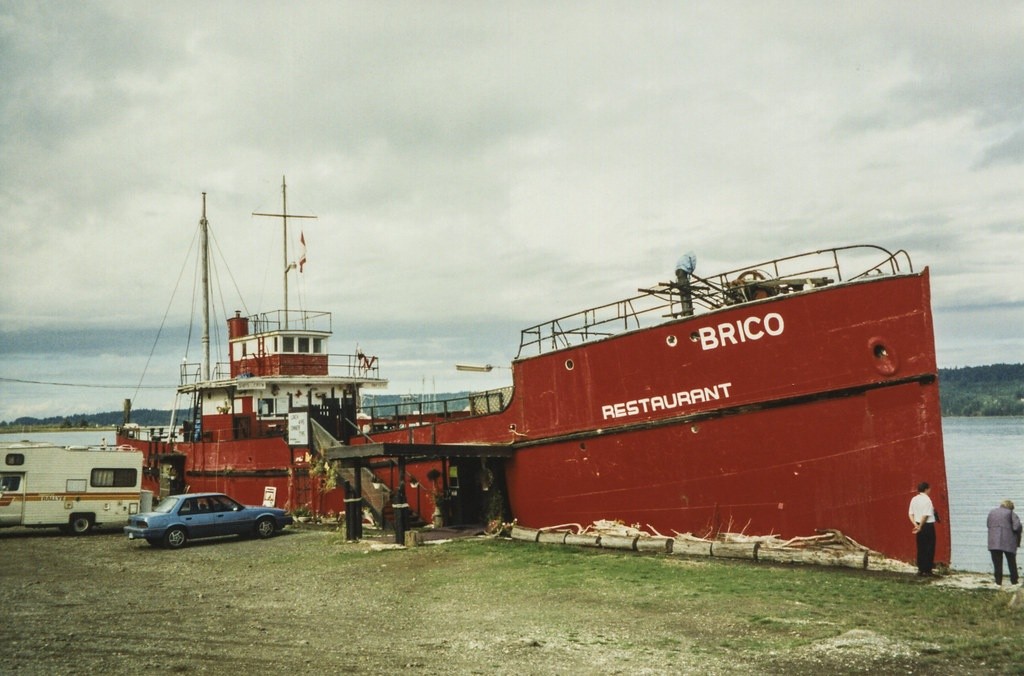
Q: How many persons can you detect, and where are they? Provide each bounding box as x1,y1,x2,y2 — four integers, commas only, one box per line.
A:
909,481,936,578
987,500,1022,585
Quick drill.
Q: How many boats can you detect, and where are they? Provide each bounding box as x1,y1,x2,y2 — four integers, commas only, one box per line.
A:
112,169,953,573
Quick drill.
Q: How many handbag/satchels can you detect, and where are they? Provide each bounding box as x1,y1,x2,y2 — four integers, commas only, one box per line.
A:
1009,509,1022,547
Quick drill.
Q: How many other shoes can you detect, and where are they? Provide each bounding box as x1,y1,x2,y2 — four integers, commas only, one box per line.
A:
995,583,1001,587
918,571,939,578
1012,583,1021,587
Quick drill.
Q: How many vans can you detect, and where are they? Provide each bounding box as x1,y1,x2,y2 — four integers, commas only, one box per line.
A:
0,441,145,535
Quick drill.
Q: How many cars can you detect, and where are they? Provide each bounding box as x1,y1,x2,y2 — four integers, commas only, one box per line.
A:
123,492,294,549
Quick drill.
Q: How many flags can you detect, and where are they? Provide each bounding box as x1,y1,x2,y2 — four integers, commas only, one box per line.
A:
298,232,306,274
